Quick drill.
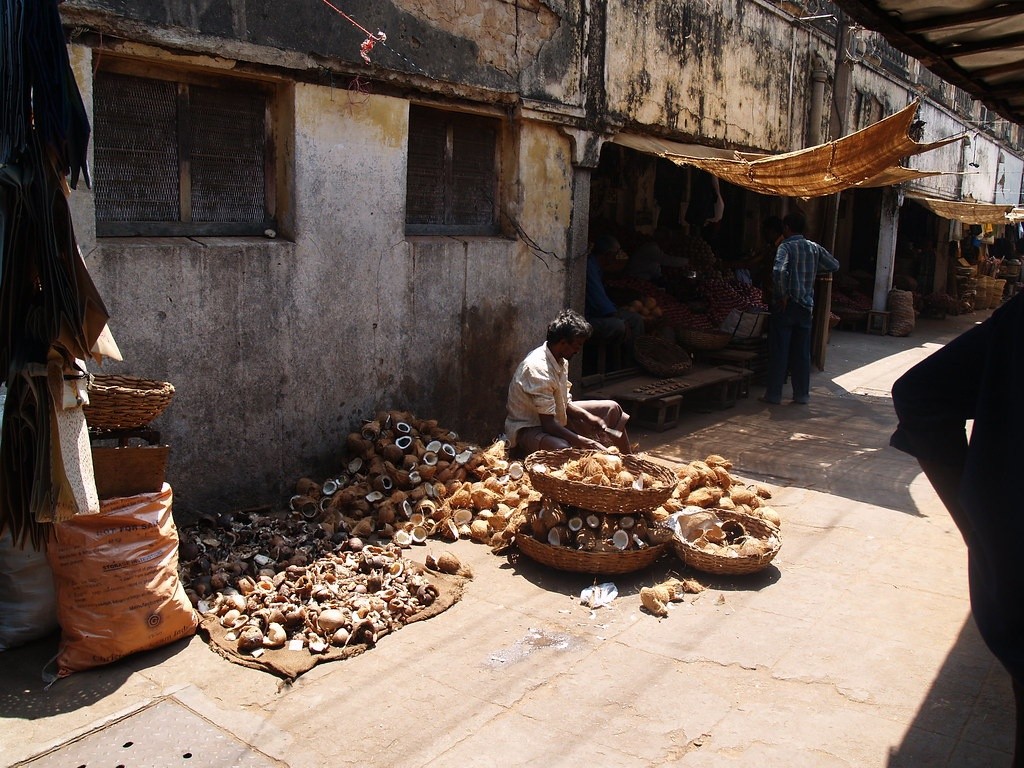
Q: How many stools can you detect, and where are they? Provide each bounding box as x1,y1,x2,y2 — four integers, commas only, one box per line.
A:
866,309,891,336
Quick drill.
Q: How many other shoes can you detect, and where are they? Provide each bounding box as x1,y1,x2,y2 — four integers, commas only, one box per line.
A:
762,396,781,404
792,396,808,404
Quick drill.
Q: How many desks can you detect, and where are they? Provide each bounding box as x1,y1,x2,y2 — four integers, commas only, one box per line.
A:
1000,274,1020,283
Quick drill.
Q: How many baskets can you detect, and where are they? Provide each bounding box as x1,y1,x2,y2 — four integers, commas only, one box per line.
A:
524,448,679,514
672,507,784,577
676,328,732,351
83,373,175,430
633,335,692,377
515,512,675,576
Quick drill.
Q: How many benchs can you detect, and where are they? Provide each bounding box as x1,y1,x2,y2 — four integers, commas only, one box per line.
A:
694,348,756,400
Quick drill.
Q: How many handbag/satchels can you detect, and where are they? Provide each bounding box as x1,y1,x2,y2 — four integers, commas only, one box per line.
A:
721,306,771,338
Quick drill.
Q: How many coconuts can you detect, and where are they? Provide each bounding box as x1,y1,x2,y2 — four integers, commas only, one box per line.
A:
287,409,780,615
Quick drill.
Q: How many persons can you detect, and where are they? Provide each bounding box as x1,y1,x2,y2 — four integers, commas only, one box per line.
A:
761,213,840,405
585,234,647,372
505,307,633,460
889,286,1024,768
